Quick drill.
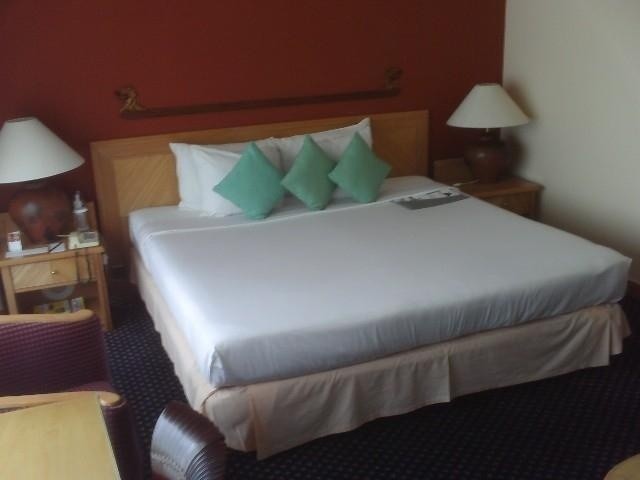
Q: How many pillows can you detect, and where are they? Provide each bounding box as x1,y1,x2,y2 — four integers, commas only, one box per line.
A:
169,116,393,222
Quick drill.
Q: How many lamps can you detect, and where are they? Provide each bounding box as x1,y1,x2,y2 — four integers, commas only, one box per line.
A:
0,116,86,245
445,82,531,183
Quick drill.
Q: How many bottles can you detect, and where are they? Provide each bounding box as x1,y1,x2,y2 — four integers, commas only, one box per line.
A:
71,190,89,235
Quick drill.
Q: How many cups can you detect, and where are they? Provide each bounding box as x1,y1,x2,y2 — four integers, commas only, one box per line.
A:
7,231,23,256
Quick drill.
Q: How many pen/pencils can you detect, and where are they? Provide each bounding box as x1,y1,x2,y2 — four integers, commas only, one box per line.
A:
49,242,63,252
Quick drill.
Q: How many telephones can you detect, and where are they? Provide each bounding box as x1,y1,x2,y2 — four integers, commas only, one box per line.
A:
67,231,100,250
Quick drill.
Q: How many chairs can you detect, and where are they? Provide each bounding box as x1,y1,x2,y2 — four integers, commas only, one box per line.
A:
0,309,144,480
149,401,229,480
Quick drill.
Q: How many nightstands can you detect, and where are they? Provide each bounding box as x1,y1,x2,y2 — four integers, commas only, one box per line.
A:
0,211,113,332
433,158,542,218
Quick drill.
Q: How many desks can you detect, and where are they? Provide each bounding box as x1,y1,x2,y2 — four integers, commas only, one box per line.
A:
0,391,123,480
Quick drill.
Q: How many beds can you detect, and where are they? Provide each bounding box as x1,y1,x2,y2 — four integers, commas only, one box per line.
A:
90,107,633,462
